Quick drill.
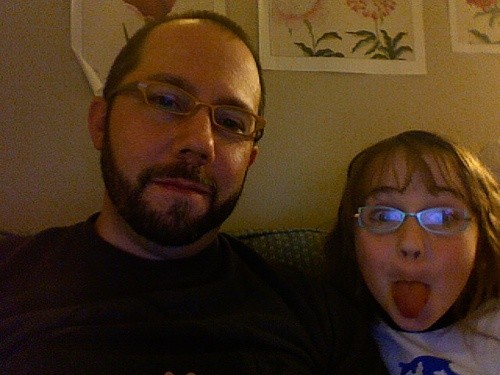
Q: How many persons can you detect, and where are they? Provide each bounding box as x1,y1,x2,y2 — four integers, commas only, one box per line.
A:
305,130,500,375
0,10,388,375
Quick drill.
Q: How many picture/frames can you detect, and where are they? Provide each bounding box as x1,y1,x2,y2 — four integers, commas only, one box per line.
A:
258,0,428,75
448,0,500,54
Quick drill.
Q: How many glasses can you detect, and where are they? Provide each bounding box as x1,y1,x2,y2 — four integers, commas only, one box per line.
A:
107,79,267,142
352,206,478,236
